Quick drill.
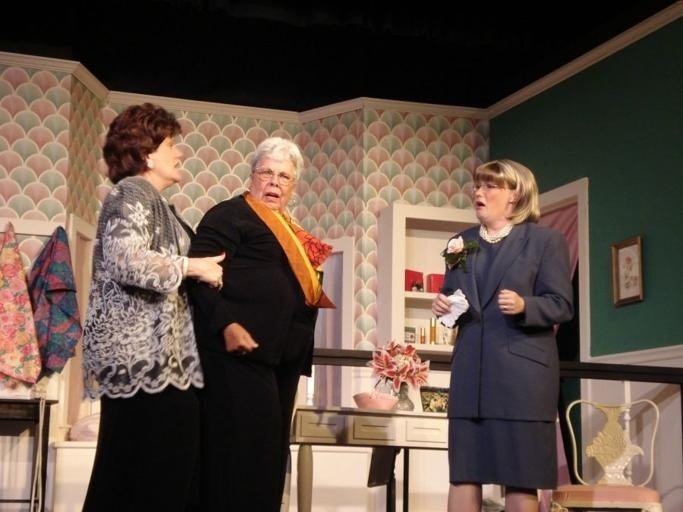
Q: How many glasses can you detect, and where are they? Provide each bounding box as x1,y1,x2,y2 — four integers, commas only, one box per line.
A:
473,184,504,192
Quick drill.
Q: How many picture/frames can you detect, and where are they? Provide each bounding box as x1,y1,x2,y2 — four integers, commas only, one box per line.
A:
611,235,645,306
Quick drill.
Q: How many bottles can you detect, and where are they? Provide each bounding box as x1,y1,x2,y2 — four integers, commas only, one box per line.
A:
411,280,424,292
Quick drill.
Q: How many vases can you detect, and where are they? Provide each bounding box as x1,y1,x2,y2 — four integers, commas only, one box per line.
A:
393,383,415,411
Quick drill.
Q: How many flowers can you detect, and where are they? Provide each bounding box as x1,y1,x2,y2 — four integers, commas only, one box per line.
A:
365,337,430,394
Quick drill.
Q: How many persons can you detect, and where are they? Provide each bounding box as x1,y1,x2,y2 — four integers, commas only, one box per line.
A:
431,159,576,511
80,102,225,508
183,137,335,512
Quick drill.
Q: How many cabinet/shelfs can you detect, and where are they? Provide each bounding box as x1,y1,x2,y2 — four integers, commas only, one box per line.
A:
51,441,506,510
378,202,483,412
1,398,59,511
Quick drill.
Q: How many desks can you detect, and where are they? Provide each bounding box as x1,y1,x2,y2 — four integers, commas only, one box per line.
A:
290,405,449,512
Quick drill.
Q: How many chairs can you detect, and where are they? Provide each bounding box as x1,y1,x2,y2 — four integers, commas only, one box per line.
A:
547,399,662,511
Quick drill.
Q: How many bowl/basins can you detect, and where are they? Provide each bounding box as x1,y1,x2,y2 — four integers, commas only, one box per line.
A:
353,392,399,411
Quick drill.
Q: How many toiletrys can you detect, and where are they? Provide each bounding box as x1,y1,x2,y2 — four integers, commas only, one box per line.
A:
419,283,424,292
431,317,437,344
420,327,425,344
411,280,418,291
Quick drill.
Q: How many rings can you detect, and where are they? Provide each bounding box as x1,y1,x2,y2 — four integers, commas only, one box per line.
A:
503,305,507,309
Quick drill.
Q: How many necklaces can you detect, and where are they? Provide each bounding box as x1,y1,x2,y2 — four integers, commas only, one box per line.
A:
479,223,513,244
